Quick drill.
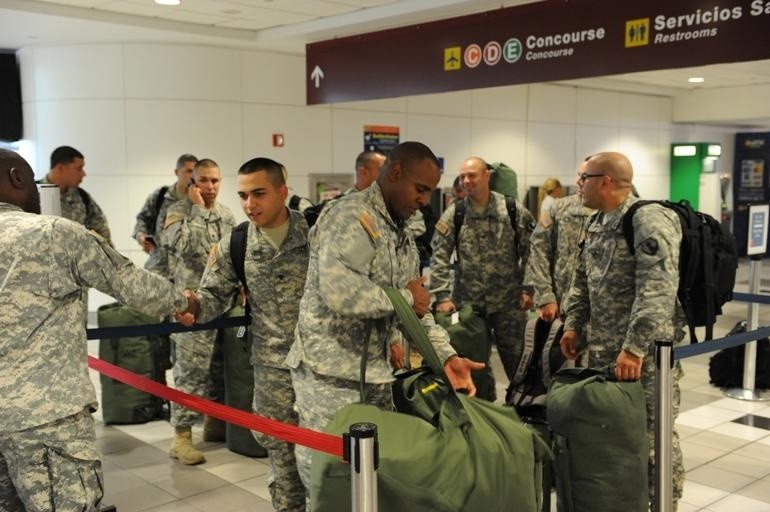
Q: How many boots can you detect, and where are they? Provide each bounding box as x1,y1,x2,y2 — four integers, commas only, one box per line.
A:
169,426,206,467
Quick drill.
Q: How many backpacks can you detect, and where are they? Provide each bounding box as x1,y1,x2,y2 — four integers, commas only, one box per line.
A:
620,199,735,326
416,203,441,267
507,317,565,397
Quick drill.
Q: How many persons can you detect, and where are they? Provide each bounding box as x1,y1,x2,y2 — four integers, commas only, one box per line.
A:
430,155,536,402
560,152,684,512
447,176,464,207
0,148,199,512
162,159,235,467
307,151,387,252
537,178,561,220
33,145,114,248
132,154,198,278
279,163,315,215
284,140,486,512
176,157,318,512
530,155,613,321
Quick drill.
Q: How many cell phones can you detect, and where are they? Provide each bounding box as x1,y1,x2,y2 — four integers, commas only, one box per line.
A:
189,178,196,185
145,236,157,248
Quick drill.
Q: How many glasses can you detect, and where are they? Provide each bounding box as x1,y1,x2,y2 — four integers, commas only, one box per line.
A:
580,173,606,181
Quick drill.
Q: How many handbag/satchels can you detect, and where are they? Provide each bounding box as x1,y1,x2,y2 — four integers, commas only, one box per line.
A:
545,363,651,512
708,334,769,392
487,162,518,200
95,299,163,425
223,303,271,458
308,284,554,512
